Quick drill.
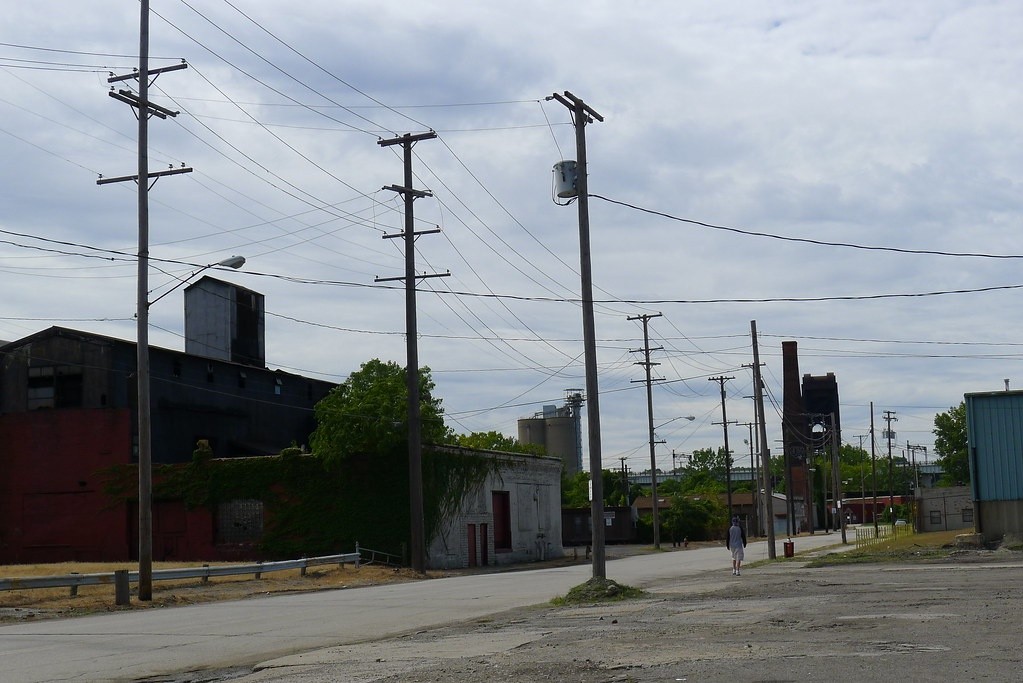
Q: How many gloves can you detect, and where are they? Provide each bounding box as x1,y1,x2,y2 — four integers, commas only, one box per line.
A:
743,544,746,548
726,546,730,550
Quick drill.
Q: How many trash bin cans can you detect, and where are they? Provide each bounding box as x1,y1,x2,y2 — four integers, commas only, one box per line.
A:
784,541,794,558
114,569,131,605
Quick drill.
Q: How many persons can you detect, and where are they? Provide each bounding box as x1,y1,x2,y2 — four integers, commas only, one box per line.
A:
726,517,747,576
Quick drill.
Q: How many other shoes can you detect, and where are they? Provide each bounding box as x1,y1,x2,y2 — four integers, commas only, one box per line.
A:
736,571,740,575
732,571,736,575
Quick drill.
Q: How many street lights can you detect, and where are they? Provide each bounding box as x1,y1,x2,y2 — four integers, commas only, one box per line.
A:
727,453,762,527
650,416,696,547
136,255,246,601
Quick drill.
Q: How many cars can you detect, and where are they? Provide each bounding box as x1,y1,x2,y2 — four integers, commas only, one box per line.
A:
895,521,906,525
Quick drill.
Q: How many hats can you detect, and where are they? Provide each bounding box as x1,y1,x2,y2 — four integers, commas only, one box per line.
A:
732,518,738,523
